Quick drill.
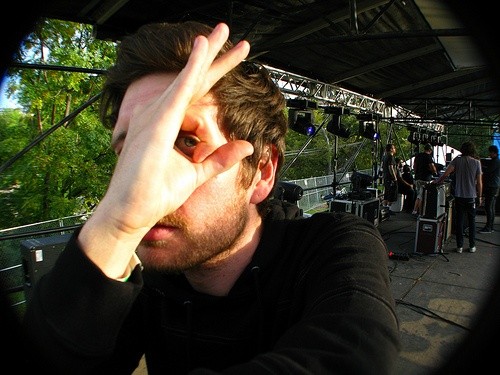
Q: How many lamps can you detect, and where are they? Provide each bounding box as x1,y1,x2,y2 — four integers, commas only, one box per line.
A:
324,106,351,138
407,126,446,147
356,114,380,140
286,99,317,136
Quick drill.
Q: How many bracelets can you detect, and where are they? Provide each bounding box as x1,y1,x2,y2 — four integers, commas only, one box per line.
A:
479,195,482,200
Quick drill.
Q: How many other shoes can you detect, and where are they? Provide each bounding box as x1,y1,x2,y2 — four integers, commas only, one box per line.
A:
417,215,423,219
470,247,476,252
412,210,417,215
457,248,462,253
479,228,494,233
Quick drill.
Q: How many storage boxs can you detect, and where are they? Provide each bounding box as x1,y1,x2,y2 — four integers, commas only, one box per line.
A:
415,213,447,255
329,197,380,228
422,185,446,219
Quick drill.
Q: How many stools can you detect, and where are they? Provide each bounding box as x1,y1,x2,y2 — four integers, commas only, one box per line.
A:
388,193,404,213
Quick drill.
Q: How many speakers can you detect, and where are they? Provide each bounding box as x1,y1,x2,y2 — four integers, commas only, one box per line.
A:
19,233,73,311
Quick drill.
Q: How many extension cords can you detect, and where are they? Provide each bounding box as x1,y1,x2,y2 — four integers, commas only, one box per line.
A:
388,251,410,261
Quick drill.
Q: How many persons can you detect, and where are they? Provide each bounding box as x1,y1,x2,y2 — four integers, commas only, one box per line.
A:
396,157,415,195
447,151,479,233
0,21,404,375
380,147,386,169
411,143,438,221
401,164,416,214
434,141,483,254
478,145,500,234
382,143,398,215
